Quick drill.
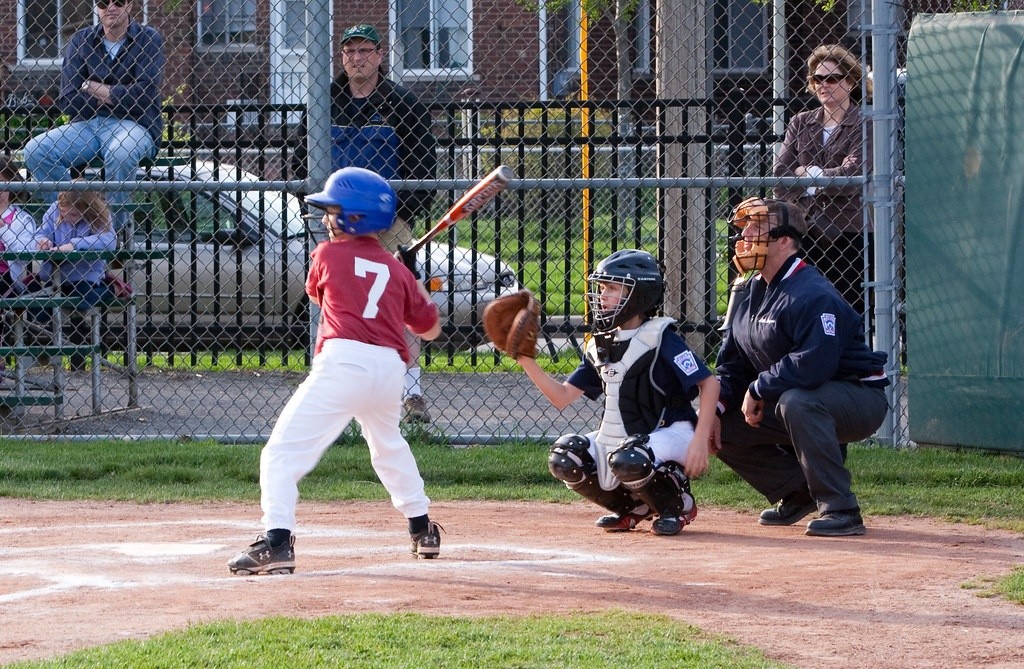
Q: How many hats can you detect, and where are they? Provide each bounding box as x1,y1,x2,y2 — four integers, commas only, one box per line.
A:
340,24,380,44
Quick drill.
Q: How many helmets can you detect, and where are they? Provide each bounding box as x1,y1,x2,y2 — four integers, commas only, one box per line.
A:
583,248,666,331
304,167,399,236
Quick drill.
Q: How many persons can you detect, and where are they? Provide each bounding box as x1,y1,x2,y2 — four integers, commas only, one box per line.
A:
0,153,36,382
23,0,162,236
291,24,436,425
693,195,891,536
228,167,441,573
771,44,874,465
514,248,720,536
27,179,117,346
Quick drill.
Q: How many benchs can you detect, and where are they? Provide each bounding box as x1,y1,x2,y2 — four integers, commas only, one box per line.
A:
0,156,198,432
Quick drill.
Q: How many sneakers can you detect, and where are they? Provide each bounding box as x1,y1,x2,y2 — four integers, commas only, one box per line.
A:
806,508,867,536
595,505,651,531
401,394,431,423
650,502,697,536
410,520,446,560
758,490,818,525
227,535,296,576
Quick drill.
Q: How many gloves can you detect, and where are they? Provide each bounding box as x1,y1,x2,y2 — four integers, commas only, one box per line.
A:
392,244,421,280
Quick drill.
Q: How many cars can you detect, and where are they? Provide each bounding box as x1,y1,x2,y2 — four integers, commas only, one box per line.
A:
17,160,517,352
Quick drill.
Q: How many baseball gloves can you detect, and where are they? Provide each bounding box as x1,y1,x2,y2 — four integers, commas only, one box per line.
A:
482,288,542,359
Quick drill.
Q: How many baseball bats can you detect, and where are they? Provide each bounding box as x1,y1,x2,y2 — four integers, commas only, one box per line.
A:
406,164,513,255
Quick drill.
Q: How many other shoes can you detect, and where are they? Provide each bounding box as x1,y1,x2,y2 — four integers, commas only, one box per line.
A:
116,225,136,243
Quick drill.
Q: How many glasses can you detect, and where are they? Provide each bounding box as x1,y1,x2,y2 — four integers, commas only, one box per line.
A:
95,0,125,9
813,73,846,84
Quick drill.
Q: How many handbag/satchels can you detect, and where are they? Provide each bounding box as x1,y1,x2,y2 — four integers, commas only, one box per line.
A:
106,273,132,299
